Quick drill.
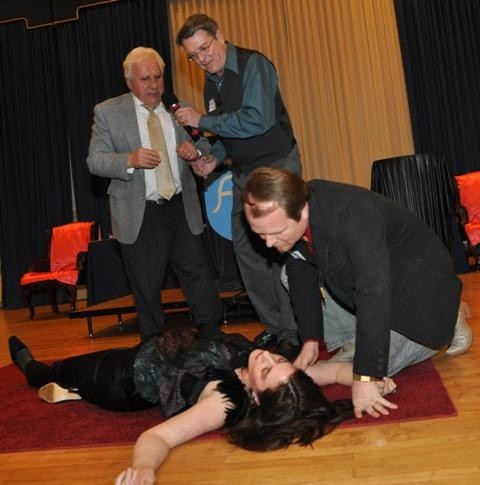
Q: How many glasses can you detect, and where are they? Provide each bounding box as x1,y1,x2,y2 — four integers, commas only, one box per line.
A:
187,36,213,61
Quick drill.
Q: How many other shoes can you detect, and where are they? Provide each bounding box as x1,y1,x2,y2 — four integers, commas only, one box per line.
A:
38,382,82,403
445,301,473,356
327,346,354,362
9,336,34,370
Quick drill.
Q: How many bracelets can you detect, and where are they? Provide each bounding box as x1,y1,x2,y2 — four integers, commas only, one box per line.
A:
353,373,383,382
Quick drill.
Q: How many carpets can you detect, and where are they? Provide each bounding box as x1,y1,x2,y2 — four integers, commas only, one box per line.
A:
0,343,457,454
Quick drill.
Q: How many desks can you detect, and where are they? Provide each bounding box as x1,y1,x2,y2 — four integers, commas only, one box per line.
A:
370,152,468,274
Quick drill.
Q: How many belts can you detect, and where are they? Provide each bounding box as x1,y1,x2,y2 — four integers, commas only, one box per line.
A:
156,193,183,205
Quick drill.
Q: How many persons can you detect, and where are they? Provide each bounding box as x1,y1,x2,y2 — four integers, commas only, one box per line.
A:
8,328,398,484
241,167,472,419
175,13,303,361
86,47,224,338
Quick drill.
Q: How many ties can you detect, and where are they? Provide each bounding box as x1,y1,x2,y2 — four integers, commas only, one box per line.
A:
142,104,177,201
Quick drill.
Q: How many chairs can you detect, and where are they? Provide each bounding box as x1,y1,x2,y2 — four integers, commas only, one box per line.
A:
18,219,99,320
454,170,480,271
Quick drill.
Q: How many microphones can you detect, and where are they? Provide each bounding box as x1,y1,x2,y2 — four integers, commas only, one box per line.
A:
161,91,200,142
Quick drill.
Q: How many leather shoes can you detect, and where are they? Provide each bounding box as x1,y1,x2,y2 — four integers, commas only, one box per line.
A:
273,340,298,360
256,331,275,343
